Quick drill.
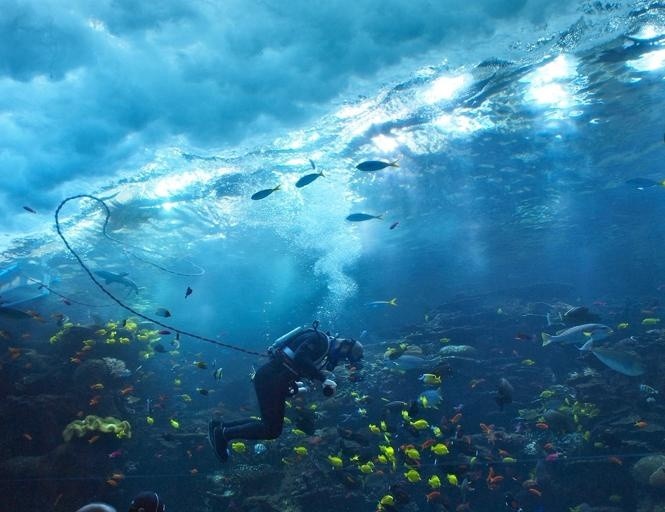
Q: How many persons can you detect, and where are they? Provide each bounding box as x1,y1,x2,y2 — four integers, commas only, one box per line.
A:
206,319,366,463
128,491,168,512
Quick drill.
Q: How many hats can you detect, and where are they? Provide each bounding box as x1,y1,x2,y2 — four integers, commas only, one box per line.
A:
129,490,168,512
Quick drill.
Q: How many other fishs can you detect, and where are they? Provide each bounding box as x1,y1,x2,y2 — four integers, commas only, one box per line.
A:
0,296,664,511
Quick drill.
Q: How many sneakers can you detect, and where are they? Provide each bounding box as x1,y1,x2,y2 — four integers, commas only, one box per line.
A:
209,421,222,450
215,427,228,462
